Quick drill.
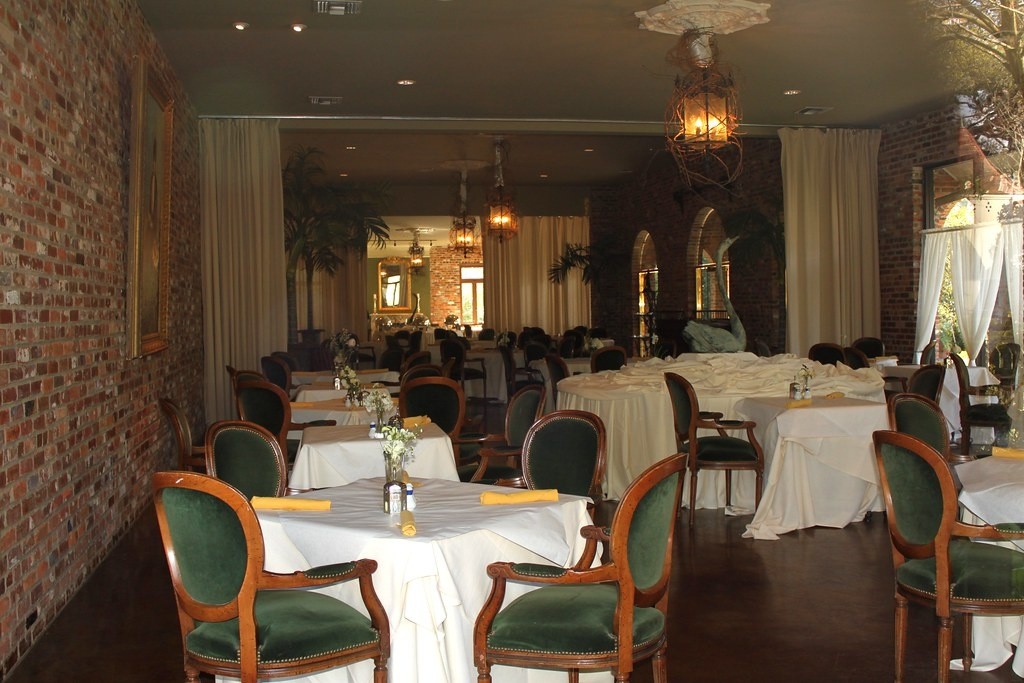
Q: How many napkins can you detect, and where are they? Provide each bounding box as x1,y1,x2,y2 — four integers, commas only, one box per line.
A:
992,446,1024,461
402,469,423,488
402,415,431,428
250,496,331,512
401,509,417,537
480,489,560,505
291,402,313,408
826,392,845,400
785,400,813,409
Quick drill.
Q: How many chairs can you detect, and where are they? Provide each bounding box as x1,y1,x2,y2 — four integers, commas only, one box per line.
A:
151,323,1024,683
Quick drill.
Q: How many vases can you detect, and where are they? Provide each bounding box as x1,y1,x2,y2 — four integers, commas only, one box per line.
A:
383,453,407,514
376,411,385,434
801,377,809,399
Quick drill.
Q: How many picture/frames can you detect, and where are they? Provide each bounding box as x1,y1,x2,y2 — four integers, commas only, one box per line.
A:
127,56,176,358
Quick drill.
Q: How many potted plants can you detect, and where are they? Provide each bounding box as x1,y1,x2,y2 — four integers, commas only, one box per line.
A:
281,143,389,349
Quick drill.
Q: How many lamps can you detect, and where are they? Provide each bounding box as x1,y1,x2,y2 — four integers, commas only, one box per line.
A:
408,232,424,267
451,166,477,258
487,141,518,244
664,27,747,205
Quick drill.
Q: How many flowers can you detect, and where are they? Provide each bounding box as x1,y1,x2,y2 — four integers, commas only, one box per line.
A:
382,424,423,456
365,382,393,413
339,366,361,395
798,364,817,378
330,329,358,359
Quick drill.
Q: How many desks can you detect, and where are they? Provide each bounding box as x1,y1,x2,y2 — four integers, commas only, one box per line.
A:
465,347,526,403
950,456,1024,678
372,328,435,361
215,475,615,683
289,369,400,402
734,395,887,539
287,397,400,440
556,352,886,517
562,357,633,377
551,335,614,351
294,382,401,401
288,423,462,490
883,365,1001,445
427,341,496,366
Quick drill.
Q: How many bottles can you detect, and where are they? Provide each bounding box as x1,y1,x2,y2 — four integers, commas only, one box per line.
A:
790,375,801,398
394,412,401,429
346,393,352,406
361,384,370,405
384,468,407,514
945,353,952,368
335,377,340,390
804,382,811,400
794,383,802,400
369,421,376,439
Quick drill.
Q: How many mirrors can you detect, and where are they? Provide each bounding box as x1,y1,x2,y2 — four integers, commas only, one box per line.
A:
378,256,412,311
